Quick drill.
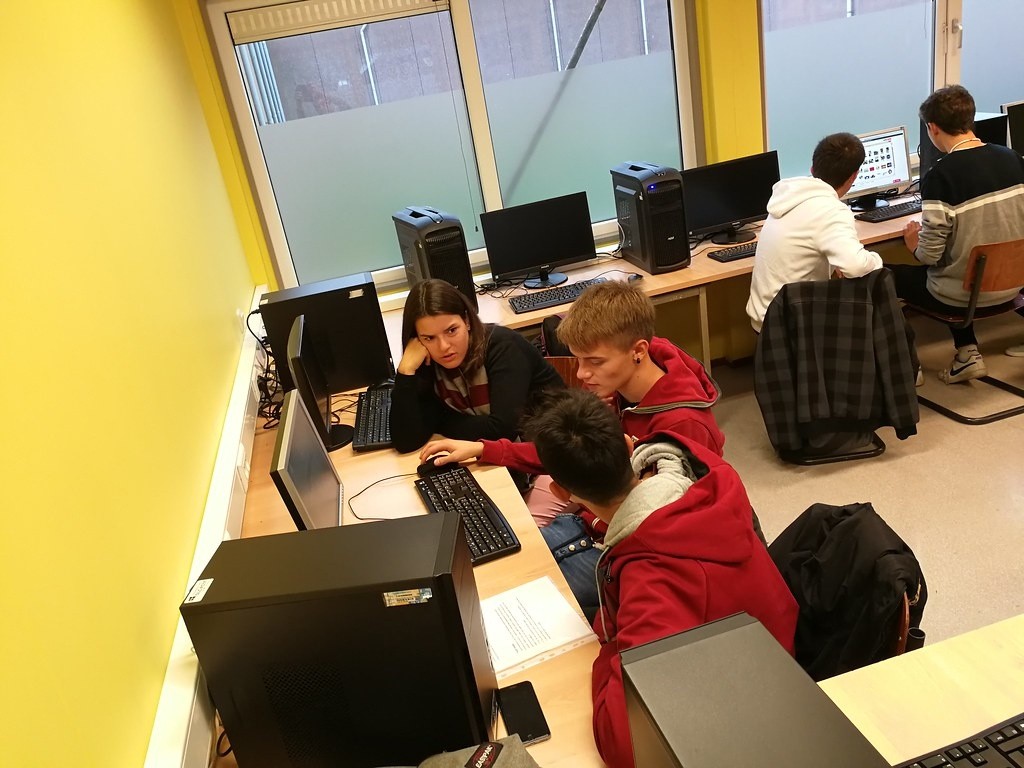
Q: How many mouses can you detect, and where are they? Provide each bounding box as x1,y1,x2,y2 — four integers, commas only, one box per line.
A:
365,377,396,392
417,455,458,477
628,273,644,284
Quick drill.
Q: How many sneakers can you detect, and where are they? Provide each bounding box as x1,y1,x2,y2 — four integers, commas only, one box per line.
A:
1004,342,1024,357
915,365,924,386
939,352,987,384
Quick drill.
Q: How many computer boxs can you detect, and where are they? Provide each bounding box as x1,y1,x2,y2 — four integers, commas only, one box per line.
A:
610,160,691,275
178,511,500,768
258,270,396,395
620,611,891,768
392,205,479,315
918,111,1008,193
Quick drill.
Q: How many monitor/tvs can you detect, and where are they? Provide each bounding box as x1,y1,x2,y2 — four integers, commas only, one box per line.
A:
839,125,912,211
480,191,597,288
681,149,781,244
270,315,355,530
1001,100,1024,158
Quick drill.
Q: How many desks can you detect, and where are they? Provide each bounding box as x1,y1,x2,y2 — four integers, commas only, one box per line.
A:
212,181,1024,768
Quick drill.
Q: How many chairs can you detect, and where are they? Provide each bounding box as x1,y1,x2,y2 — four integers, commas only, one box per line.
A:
768,504,920,681
756,268,895,465
899,239,1024,425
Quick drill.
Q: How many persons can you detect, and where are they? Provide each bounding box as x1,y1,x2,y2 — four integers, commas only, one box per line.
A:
517,385,800,768
418,278,725,607
883,84,1024,388
745,132,883,337
388,279,571,530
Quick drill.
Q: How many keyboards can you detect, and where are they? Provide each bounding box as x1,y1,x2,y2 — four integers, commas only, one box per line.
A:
352,390,396,453
414,465,521,567
853,199,923,223
508,277,611,314
707,241,758,263
891,712,1024,768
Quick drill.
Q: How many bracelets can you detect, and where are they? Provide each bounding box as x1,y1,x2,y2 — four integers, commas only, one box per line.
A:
913,248,921,262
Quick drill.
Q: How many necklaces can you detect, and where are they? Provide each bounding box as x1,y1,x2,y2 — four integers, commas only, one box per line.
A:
948,138,981,154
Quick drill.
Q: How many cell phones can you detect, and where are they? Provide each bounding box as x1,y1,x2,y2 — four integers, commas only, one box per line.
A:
496,681,551,747
